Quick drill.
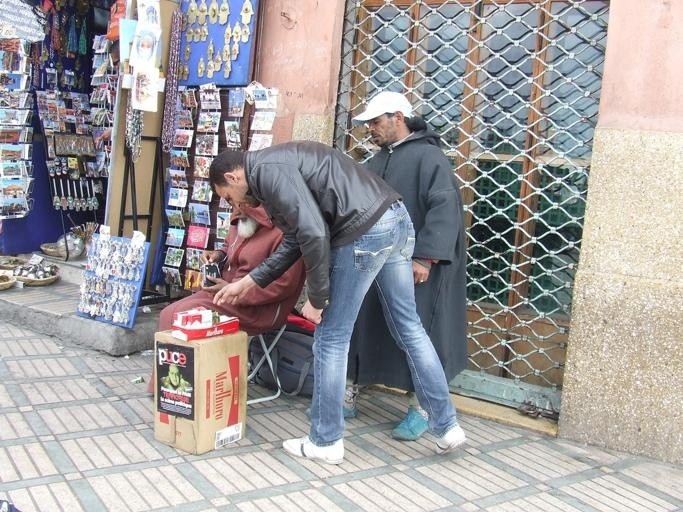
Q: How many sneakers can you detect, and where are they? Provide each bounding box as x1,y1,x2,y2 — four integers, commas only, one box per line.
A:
281,435,346,466
352,91,412,125
305,404,359,420
392,406,428,442
432,425,468,456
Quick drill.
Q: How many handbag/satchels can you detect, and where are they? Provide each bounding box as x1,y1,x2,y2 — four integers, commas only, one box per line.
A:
244,327,321,400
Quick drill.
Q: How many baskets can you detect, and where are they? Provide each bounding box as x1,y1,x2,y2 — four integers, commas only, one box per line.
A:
0,272,16,291
14,270,59,287
40,242,62,256
0,255,25,270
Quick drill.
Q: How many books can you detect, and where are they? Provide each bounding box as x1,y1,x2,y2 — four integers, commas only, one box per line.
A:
161,87,247,291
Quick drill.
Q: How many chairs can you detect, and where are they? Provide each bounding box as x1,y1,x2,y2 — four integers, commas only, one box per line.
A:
238,255,305,407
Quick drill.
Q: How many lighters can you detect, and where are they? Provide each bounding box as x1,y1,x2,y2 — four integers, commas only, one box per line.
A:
212,309,219,326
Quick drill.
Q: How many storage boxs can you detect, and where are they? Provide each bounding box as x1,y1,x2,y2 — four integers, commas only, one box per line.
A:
149,327,249,456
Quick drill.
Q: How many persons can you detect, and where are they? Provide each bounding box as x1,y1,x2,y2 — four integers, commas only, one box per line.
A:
210,141,466,464
148,200,309,395
160,363,191,396
307,91,468,442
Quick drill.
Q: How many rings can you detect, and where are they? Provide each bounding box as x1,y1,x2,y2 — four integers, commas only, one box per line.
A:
418,280,424,284
